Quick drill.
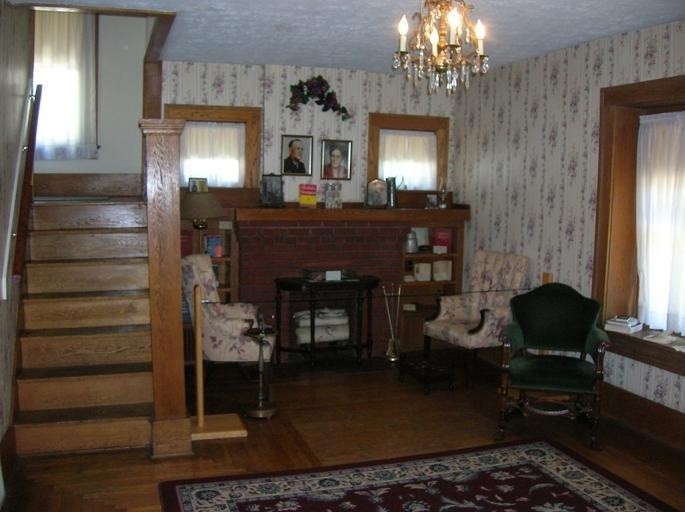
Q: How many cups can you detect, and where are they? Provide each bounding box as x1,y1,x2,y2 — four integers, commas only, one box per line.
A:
414,263,431,282
426,195,438,210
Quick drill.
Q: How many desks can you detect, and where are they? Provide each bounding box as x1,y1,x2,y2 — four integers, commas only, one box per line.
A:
274,275,380,376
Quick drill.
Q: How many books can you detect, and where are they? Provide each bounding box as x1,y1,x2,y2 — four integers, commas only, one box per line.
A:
604,315,643,335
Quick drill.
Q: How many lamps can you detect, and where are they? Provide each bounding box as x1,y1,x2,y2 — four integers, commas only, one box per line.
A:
391,0,491,98
181,192,226,254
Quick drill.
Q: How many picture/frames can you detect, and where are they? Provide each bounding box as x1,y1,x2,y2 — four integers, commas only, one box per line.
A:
366,178,388,209
321,139,352,180
281,134,313,176
261,172,284,207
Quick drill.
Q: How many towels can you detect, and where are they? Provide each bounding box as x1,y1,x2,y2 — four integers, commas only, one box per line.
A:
292,308,350,345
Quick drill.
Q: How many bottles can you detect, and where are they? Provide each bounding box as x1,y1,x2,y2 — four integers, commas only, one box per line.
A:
386,177,396,208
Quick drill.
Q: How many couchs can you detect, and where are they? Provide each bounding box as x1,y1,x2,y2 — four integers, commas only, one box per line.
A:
180,253,277,421
492,282,611,452
419,250,531,394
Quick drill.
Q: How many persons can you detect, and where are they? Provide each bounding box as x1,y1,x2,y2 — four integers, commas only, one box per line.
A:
284,139,306,173
324,144,348,178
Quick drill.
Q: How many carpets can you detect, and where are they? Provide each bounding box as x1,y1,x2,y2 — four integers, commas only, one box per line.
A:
158,436,680,511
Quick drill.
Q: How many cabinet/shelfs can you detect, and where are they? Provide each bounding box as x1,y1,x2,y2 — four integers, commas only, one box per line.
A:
193,206,470,363
